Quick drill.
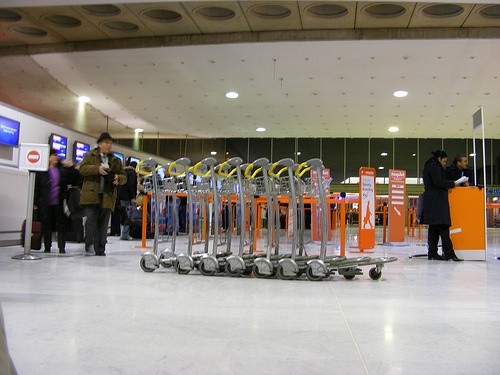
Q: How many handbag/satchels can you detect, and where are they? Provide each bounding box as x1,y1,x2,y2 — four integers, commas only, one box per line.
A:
417,195,425,224
20,218,44,250
63,186,83,220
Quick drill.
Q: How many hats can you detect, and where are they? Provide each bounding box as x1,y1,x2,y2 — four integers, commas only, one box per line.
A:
97,133,116,143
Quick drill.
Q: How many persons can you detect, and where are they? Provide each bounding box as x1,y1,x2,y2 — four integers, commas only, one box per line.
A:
422,150,464,261
78,133,127,256
34,149,74,253
445,154,473,186
118,161,187,241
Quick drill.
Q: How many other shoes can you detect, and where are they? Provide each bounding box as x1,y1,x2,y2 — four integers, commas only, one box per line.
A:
59,247,65,253
43,247,51,253
85,245,96,255
95,251,106,256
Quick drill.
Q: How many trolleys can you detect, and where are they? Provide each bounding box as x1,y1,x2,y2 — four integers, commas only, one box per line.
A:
135,155,398,281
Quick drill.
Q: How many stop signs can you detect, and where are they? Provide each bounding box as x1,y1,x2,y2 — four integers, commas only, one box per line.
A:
27,151,40,163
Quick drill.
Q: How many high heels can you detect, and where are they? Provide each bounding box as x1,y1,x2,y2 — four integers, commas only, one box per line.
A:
444,256,465,260
428,254,445,261
